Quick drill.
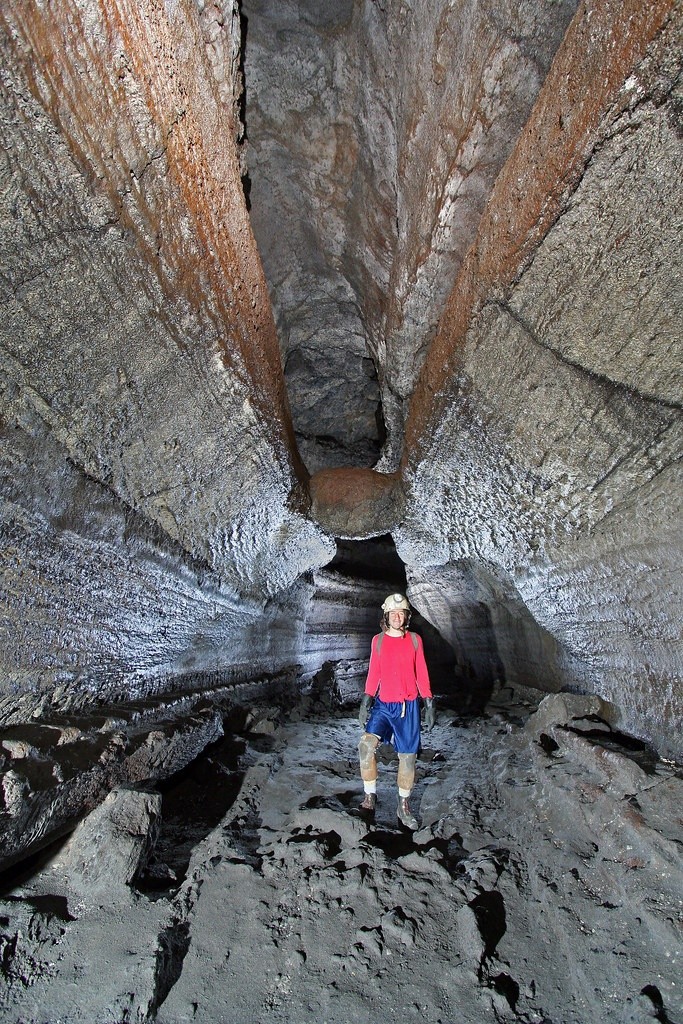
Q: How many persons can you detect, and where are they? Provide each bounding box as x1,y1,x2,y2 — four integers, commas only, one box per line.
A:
348,592,433,831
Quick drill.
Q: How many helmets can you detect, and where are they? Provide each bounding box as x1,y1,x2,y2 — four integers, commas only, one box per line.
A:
381,594,410,638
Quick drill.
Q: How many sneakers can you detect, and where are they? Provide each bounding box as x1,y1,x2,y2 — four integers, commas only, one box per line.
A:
397,796,418,830
349,793,377,818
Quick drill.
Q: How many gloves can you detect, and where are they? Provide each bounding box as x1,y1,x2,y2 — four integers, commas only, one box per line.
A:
359,694,372,729
425,699,436,732
